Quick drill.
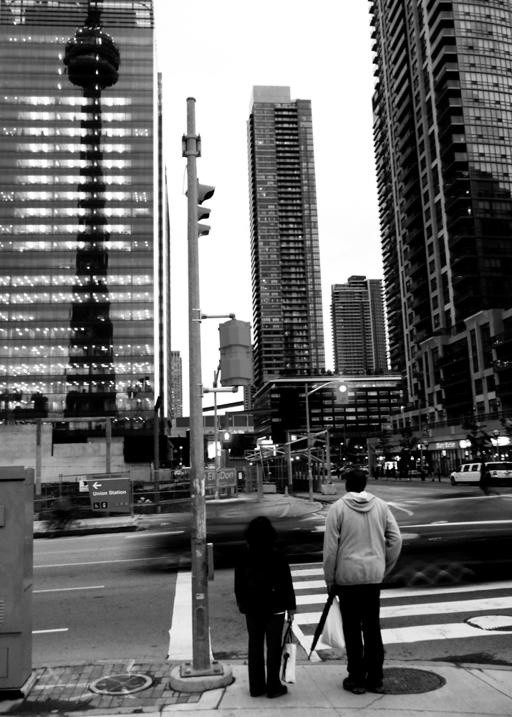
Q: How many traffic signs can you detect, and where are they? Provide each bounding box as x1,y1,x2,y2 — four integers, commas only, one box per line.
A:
87,478,135,512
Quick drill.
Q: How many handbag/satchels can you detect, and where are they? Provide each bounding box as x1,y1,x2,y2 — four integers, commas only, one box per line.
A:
282,643,296,684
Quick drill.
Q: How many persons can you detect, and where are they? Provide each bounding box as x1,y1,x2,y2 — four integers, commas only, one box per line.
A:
321,469,405,694
232,514,297,699
335,463,435,482
476,462,493,495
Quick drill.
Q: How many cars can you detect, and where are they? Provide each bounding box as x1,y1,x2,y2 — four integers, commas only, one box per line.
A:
311,463,370,479
448,460,512,486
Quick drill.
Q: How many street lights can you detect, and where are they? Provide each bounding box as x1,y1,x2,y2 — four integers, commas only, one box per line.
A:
491,428,500,460
201,388,238,470
339,441,346,464
423,440,431,481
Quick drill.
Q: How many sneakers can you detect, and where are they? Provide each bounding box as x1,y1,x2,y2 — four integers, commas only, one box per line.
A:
250,683,287,697
343,678,384,694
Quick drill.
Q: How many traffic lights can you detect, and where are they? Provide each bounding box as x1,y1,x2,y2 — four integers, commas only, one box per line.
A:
192,181,215,238
337,382,350,405
220,431,232,442
